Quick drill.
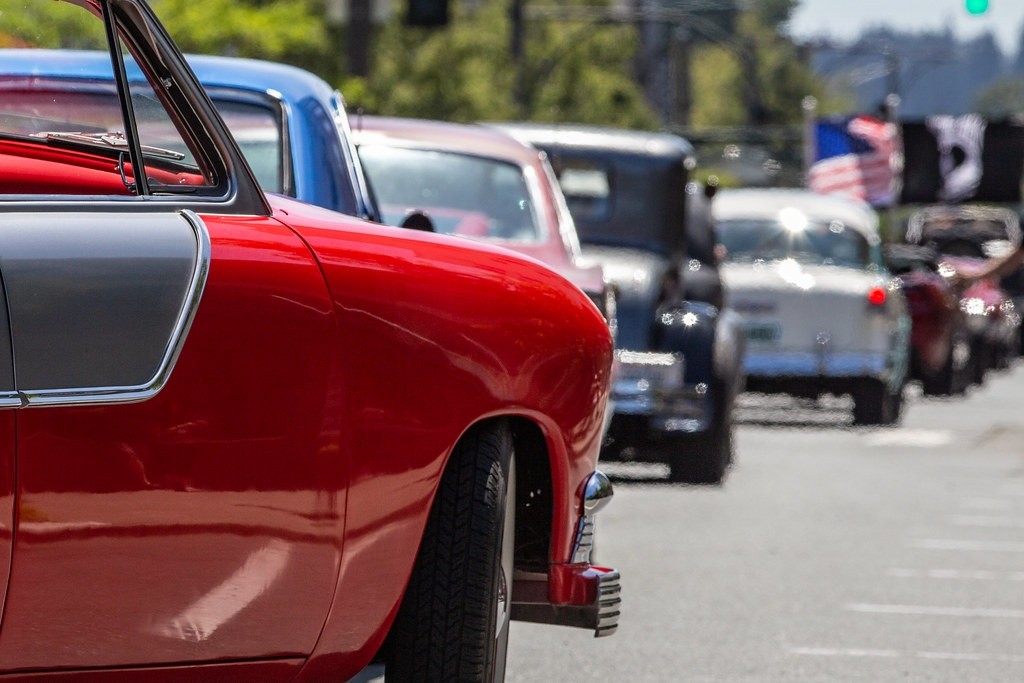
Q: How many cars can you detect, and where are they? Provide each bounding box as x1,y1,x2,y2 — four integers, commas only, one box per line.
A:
438,121,750,484
0,1,622,683
0,45,384,229
708,184,915,426
886,196,1024,397
111,108,616,360
